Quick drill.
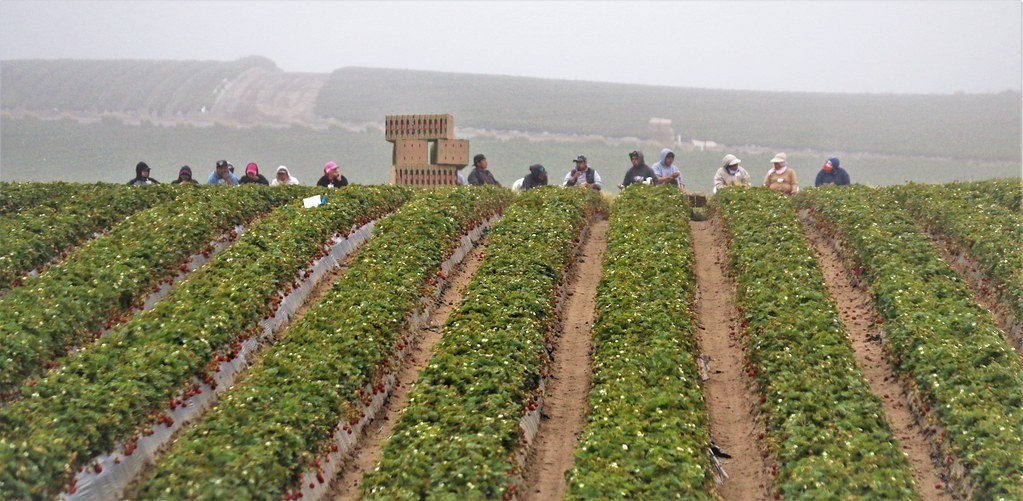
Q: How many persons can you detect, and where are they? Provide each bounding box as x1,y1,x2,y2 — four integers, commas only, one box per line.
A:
271,166,301,187
650,147,685,190
237,163,269,186
130,161,161,187
468,154,500,187
815,157,851,187
206,159,241,186
172,165,201,186
617,151,661,189
563,153,602,191
764,153,798,195
520,162,548,190
316,161,350,188
713,154,752,195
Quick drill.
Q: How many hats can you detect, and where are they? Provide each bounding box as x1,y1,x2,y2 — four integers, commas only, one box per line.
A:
769,152,788,163
246,164,257,174
216,160,227,168
226,161,233,169
573,155,586,162
629,151,638,158
532,167,547,174
722,154,742,168
822,160,833,171
278,168,286,174
180,169,191,176
324,161,340,176
471,154,485,166
142,166,152,170
667,152,674,159
